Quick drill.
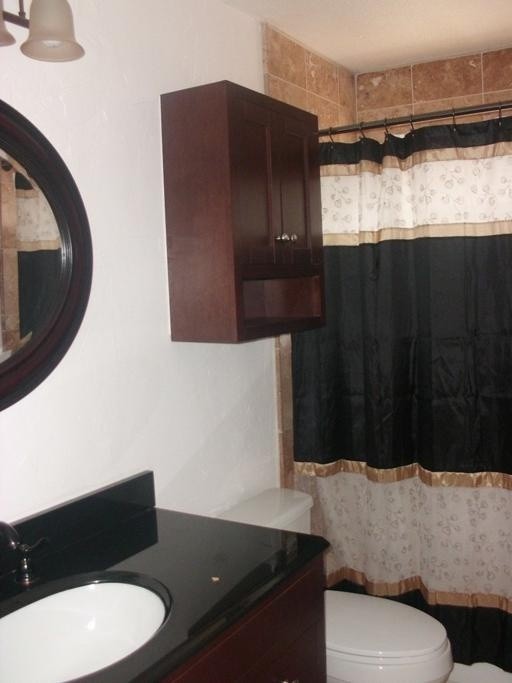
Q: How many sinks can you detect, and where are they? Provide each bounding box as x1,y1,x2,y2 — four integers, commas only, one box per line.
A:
0,570,175,683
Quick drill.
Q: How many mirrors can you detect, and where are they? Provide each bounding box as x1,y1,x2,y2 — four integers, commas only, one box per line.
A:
0,100,94,415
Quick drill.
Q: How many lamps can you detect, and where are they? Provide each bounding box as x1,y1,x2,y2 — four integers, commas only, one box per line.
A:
0,1,84,63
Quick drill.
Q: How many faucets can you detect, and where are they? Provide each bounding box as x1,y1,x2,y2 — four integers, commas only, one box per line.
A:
0,521,23,552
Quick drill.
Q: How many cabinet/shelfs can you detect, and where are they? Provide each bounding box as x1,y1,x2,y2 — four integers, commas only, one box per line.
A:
161,80,325,342
161,553,327,683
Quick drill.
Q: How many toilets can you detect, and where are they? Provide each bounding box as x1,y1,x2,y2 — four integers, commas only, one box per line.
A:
214,484,455,683
